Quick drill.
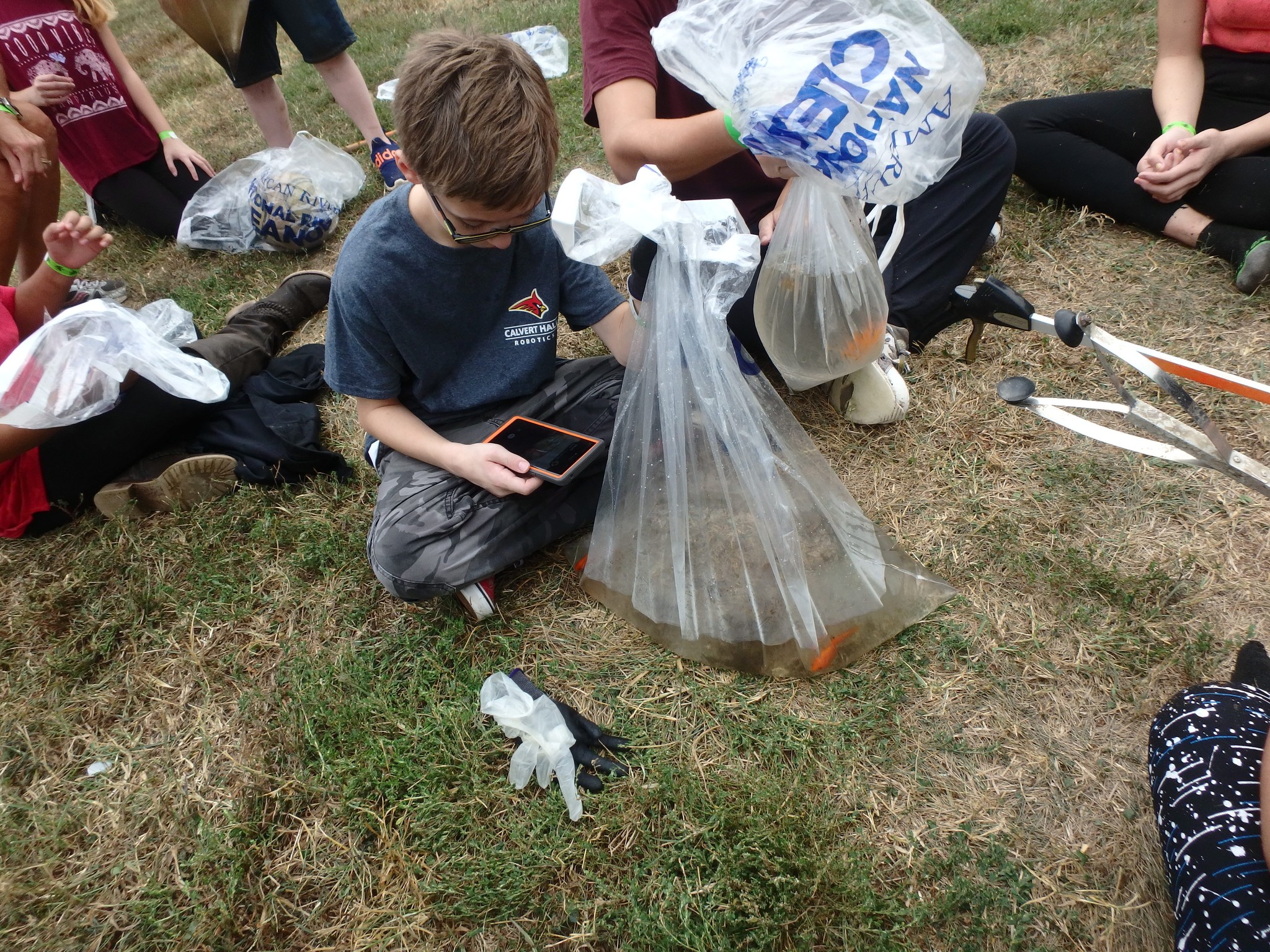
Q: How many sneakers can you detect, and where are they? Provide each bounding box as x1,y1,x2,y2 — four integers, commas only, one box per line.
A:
59,279,130,314
454,559,525,622
85,193,111,227
370,128,408,192
820,323,912,425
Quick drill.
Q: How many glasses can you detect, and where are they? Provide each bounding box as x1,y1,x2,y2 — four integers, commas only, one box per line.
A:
424,181,553,245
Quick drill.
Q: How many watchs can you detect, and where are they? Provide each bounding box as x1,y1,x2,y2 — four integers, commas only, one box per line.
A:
0,96,23,121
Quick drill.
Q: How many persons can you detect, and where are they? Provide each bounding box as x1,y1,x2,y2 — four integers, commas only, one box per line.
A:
320,29,647,624
1,1,216,238
994,2,1270,298
579,0,1016,424
2,211,329,539
1144,642,1270,952
1,64,129,307
214,1,408,191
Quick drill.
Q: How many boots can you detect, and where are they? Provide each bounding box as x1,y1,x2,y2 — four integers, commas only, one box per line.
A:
178,269,332,395
93,443,240,522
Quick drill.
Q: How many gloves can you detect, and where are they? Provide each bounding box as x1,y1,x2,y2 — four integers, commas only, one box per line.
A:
509,668,633,793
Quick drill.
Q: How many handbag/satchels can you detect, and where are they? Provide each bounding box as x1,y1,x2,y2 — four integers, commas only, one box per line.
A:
548,164,955,680
0,297,232,430
649,0,988,393
174,131,366,254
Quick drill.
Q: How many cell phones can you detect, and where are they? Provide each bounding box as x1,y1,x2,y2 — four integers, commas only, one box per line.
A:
479,414,605,486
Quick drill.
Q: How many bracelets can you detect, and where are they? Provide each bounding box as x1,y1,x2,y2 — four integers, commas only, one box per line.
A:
158,131,178,141
43,251,81,276
0,104,13,113
724,113,750,149
1163,121,1197,134
